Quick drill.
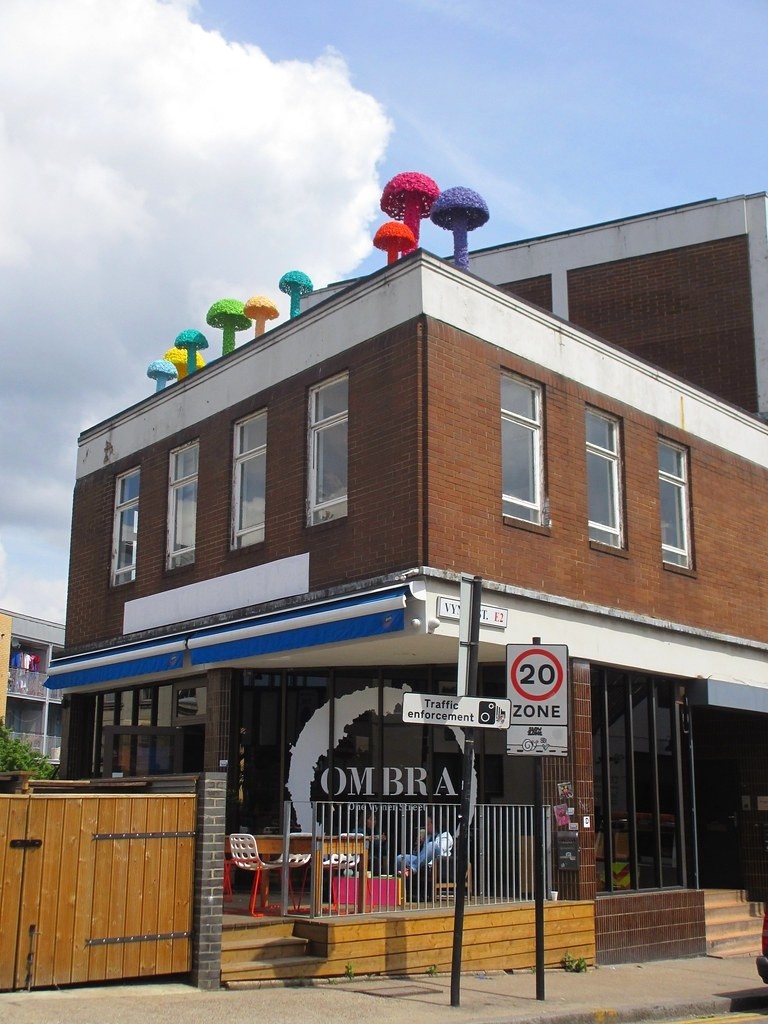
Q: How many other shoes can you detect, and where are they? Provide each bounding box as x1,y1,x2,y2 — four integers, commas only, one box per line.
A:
398,867,416,879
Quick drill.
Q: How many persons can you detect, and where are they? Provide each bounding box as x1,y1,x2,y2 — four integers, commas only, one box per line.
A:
352,810,388,876
397,811,455,877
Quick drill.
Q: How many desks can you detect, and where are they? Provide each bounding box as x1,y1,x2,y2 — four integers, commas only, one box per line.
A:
225,833,386,917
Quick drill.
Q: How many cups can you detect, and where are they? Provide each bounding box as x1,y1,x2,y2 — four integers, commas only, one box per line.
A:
551,890,558,904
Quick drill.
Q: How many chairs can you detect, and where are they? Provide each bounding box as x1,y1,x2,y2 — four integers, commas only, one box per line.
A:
295,833,376,916
413,827,480,902
223,833,312,917
